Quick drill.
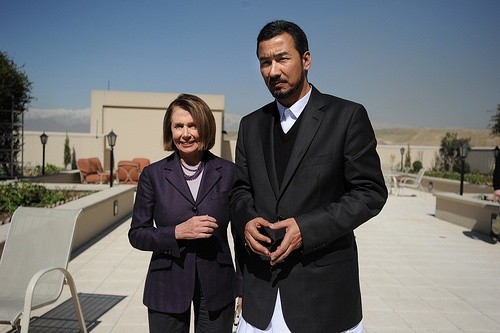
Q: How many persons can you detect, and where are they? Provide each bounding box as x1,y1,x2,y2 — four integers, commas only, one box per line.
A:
128,92,237,333
493,150,500,201
231,20,389,333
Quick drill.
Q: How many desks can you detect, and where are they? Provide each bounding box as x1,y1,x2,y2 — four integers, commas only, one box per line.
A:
383,173,401,195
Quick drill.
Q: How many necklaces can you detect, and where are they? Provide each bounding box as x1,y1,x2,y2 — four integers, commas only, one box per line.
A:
182,161,203,180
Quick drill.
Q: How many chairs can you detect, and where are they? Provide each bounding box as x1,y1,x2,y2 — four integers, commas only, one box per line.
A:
397,168,426,197
0,206,87,333
77,157,150,186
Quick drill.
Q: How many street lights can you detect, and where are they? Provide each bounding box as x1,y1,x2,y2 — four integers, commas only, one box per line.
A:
459,141,469,195
40,131,48,176
107,129,117,187
400,147,405,171
